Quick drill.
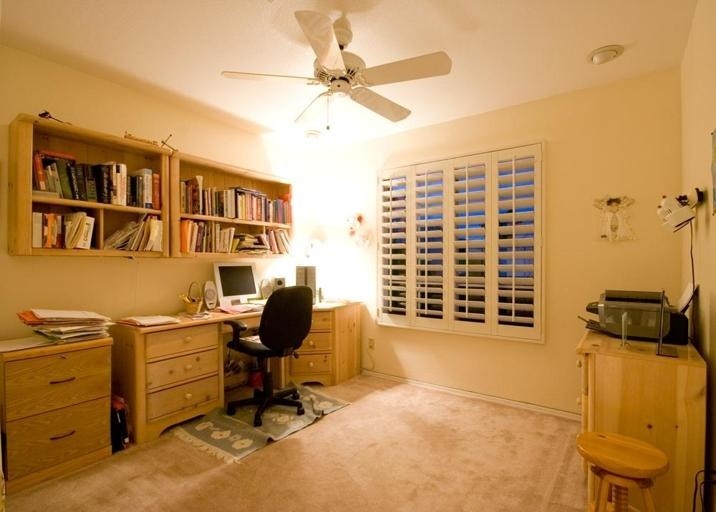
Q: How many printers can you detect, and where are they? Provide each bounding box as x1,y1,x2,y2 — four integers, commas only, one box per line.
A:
577,289,688,345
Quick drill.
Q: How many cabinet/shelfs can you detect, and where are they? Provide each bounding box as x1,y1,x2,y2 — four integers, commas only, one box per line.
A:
171,150,292,258
573,329,707,512
0,334,114,496
7,113,171,258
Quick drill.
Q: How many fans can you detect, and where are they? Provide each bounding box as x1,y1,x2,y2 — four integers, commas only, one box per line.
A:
220,5,453,125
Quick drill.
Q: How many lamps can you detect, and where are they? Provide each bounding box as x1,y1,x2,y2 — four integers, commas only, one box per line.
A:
656,187,707,339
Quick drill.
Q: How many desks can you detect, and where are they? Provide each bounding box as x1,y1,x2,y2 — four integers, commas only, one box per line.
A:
116,298,363,446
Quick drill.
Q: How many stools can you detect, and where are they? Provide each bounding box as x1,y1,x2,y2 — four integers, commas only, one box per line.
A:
572,431,670,512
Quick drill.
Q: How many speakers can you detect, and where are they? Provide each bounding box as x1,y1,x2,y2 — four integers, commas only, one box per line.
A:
202,280,218,310
273,277,285,292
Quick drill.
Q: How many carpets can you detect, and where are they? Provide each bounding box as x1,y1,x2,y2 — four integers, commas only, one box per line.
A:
170,381,353,465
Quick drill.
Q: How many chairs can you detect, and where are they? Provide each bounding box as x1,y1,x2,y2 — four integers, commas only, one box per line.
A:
222,285,315,428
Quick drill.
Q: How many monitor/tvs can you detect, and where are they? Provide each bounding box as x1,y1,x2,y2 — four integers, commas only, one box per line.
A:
213,261,262,304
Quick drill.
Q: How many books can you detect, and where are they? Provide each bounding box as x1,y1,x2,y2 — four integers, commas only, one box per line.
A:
17,306,114,345
31,149,292,256
118,314,180,328
192,312,211,320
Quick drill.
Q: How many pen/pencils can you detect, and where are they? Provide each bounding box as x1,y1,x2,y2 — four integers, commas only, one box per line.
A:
179,295,201,303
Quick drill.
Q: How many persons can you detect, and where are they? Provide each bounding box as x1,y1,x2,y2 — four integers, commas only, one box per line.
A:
593,192,637,243
